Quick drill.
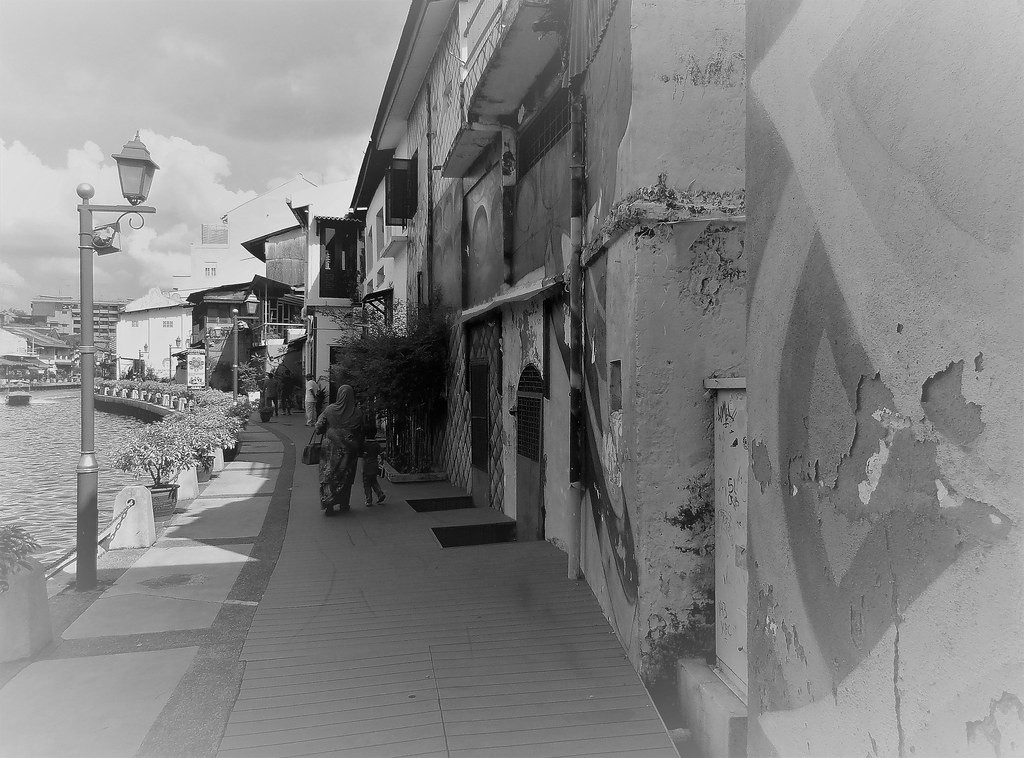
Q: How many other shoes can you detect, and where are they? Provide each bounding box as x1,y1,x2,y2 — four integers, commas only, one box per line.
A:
376,495,386,503
340,505,350,511
365,501,372,506
324,508,334,515
276,413,279,416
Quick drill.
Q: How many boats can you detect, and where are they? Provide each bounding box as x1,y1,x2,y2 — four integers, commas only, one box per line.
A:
6,380,32,406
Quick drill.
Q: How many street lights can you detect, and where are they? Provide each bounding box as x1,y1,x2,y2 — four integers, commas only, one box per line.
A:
137,343,149,383
169,336,182,386
74,131,158,593
232,291,260,407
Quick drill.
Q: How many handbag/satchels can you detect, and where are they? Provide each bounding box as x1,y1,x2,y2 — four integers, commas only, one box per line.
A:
301,429,322,465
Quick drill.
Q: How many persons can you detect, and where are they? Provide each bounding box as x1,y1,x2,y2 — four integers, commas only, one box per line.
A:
303,373,320,426
262,370,295,416
359,425,386,506
315,385,367,515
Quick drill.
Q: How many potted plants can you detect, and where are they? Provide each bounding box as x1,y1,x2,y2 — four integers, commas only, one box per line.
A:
111,420,194,522
163,411,233,482
238,352,288,422
206,410,249,462
